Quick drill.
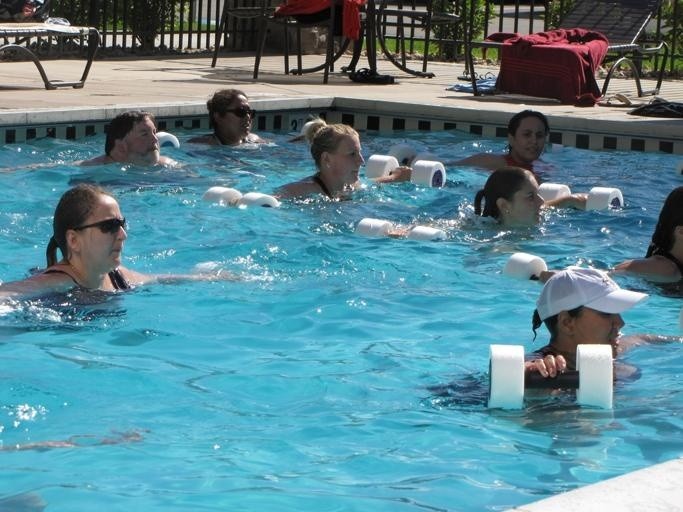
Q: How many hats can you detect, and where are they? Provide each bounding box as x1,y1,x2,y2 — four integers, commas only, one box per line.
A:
535,265,651,321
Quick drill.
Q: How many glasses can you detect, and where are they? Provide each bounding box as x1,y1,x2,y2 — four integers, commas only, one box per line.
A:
72,217,127,235
220,106,257,121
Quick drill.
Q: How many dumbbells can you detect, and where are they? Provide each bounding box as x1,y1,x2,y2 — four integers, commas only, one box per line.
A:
357,218,446,242
488,345,613,411
366,154,446,188
540,183,624,211
202,186,279,209
388,144,440,167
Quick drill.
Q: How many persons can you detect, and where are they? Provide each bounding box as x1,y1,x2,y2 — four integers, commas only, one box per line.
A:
0,110,239,188
185,89,318,145
539,187,683,298
428,266,683,412
0,429,146,512
0,183,258,311
388,166,589,239
228,118,412,207
407,110,554,178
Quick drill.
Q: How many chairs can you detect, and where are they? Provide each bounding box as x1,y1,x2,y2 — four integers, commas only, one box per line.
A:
0,1,101,90
212,0,300,77
359,1,470,76
468,0,669,104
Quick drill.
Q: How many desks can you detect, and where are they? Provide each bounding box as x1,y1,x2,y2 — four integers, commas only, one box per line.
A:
289,0,435,82
